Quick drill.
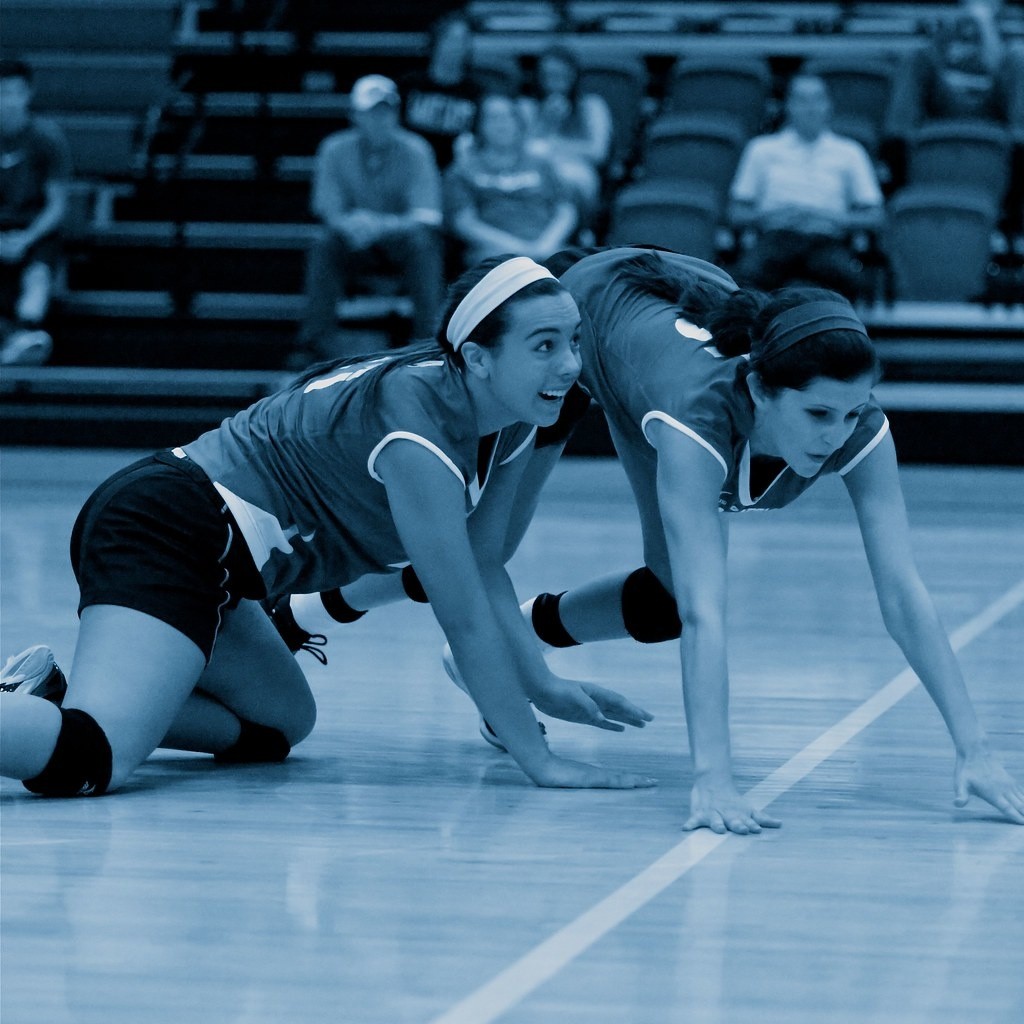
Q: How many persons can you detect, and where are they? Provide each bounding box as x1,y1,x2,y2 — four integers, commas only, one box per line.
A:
1,0,1023,365
0,251,660,797
258,246,1024,838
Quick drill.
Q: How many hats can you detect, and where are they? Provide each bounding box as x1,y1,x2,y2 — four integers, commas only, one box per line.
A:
348,74,402,110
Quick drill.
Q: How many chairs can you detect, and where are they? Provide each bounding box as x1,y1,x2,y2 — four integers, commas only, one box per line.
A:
642,113,747,195
909,123,1009,208
807,59,903,132
669,61,773,133
571,50,648,177
614,182,719,262
890,193,995,303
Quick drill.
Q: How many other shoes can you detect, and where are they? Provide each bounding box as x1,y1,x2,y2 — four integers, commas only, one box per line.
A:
0,641,70,708
1,324,55,367
438,641,549,751
